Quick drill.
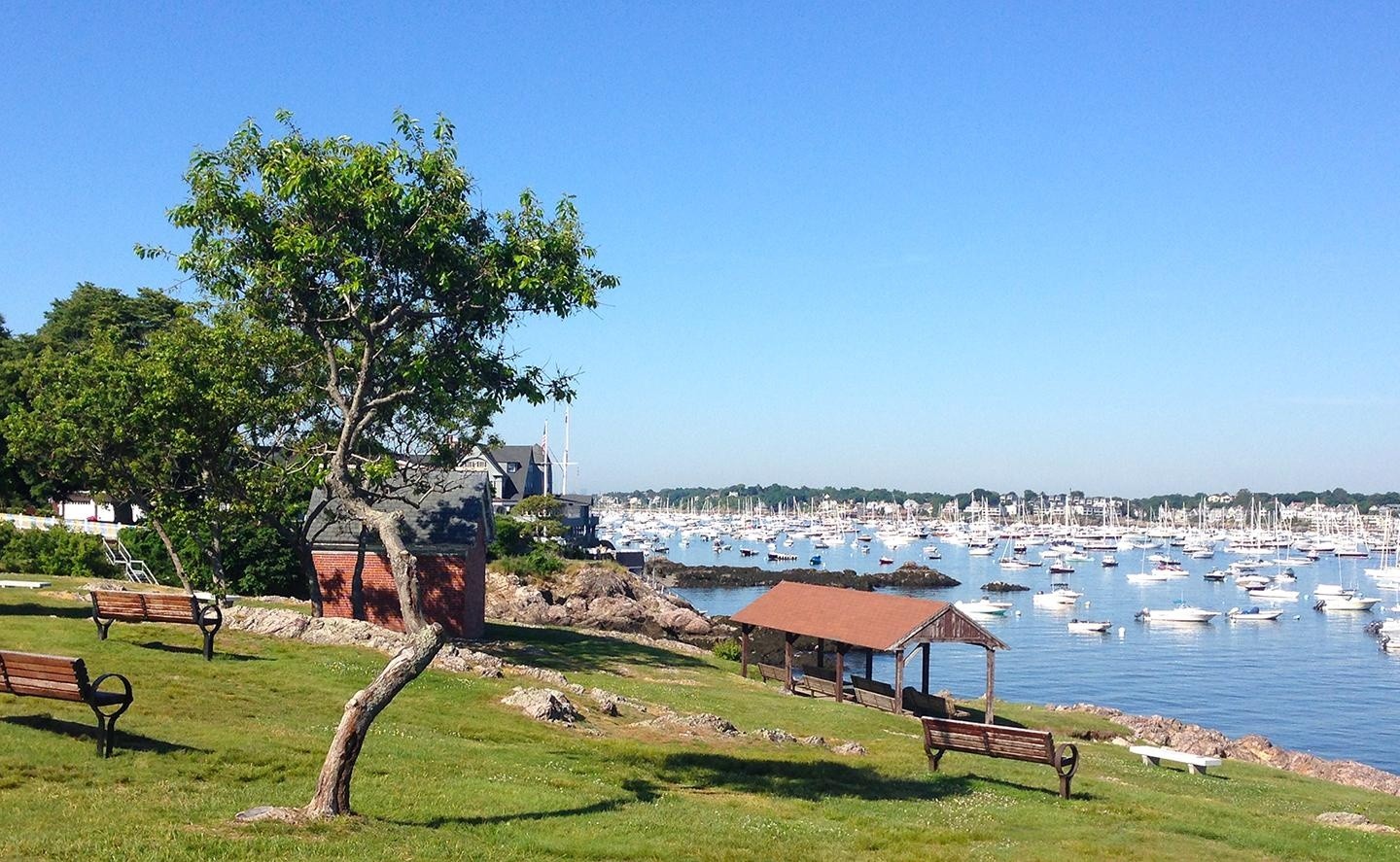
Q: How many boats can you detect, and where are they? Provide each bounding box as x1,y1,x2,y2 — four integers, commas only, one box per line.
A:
592,496,1400,663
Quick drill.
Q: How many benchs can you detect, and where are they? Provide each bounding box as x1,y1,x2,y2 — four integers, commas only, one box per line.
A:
1129,745,1222,776
910,690,949,719
854,688,896,714
757,662,798,693
192,591,241,609
0,579,52,589
802,674,837,700
0,650,134,760
90,588,223,662
849,674,895,698
921,715,1080,801
802,663,836,682
